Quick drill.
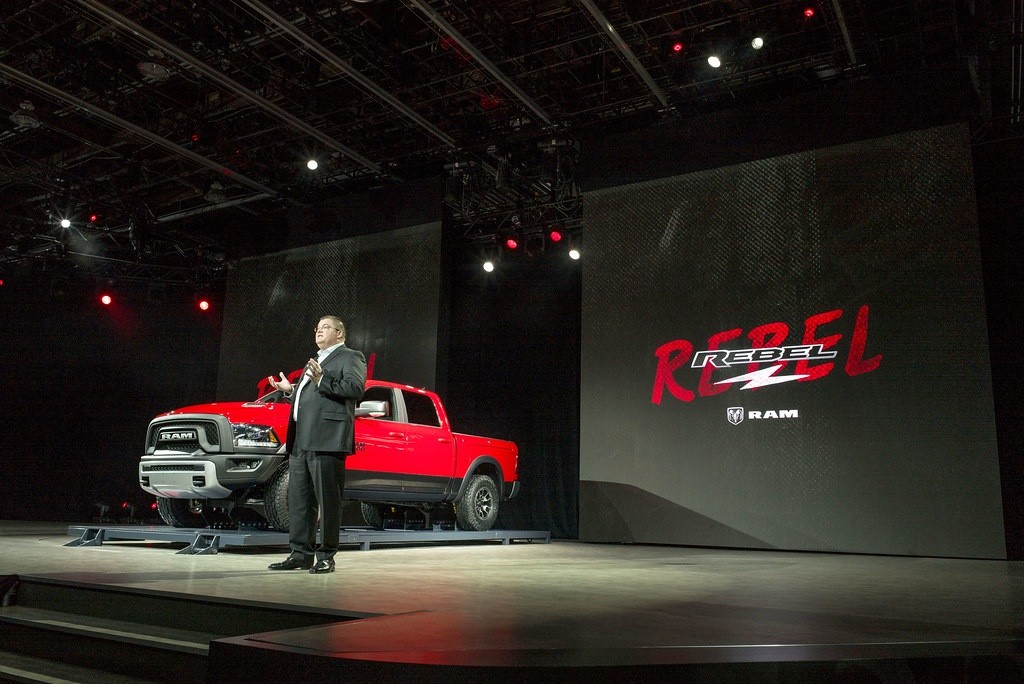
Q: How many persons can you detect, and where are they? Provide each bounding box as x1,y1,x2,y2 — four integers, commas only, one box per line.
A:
267,316,366,574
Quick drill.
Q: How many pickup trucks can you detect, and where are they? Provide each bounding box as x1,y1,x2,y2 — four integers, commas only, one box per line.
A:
139,380,520,532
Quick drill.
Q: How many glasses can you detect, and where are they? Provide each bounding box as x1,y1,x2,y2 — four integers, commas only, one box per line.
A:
314,325,342,333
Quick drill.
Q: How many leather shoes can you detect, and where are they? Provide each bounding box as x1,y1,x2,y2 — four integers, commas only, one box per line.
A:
309,558,335,574
267,553,313,570
